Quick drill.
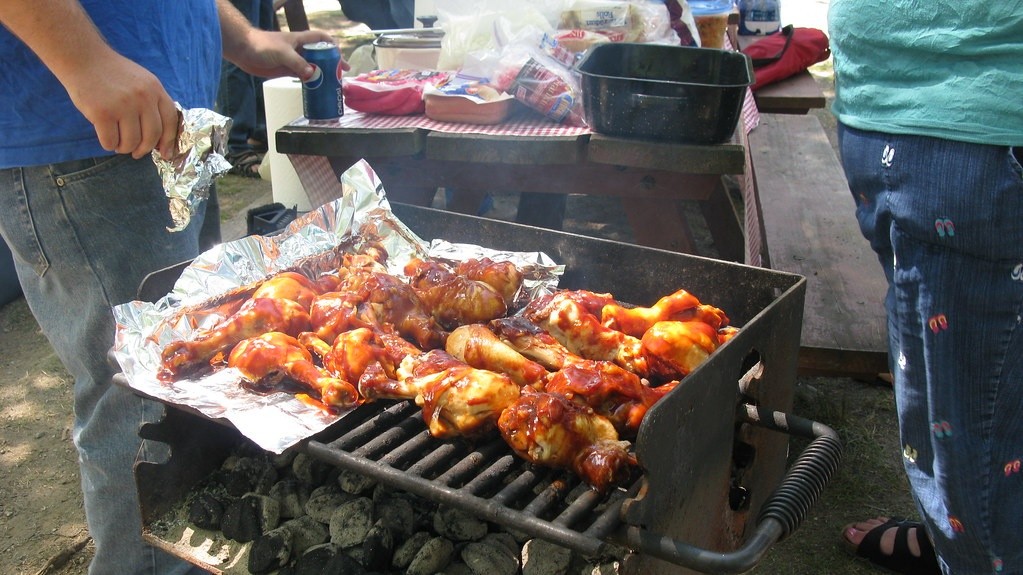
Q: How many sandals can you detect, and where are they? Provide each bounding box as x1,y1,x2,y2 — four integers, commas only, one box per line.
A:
841,517,940,575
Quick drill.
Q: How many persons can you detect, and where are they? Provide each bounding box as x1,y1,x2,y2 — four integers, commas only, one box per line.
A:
0,0,343,574
828,0,1023,575
215,0,286,176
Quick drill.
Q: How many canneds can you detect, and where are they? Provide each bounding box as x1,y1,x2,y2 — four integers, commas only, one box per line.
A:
303,40,344,124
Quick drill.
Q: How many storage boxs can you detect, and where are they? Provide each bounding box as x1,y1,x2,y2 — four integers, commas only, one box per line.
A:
574,41,757,144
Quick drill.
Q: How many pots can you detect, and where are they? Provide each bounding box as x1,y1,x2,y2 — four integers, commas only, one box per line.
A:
371,16,448,72
574,41,756,145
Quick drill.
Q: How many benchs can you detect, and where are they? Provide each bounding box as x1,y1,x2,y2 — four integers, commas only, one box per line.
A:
737,34,826,115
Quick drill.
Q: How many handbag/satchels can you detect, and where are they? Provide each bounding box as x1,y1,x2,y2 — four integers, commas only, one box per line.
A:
744,24,830,88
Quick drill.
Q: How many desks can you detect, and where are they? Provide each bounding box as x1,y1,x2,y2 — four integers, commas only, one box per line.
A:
276,0,772,271
